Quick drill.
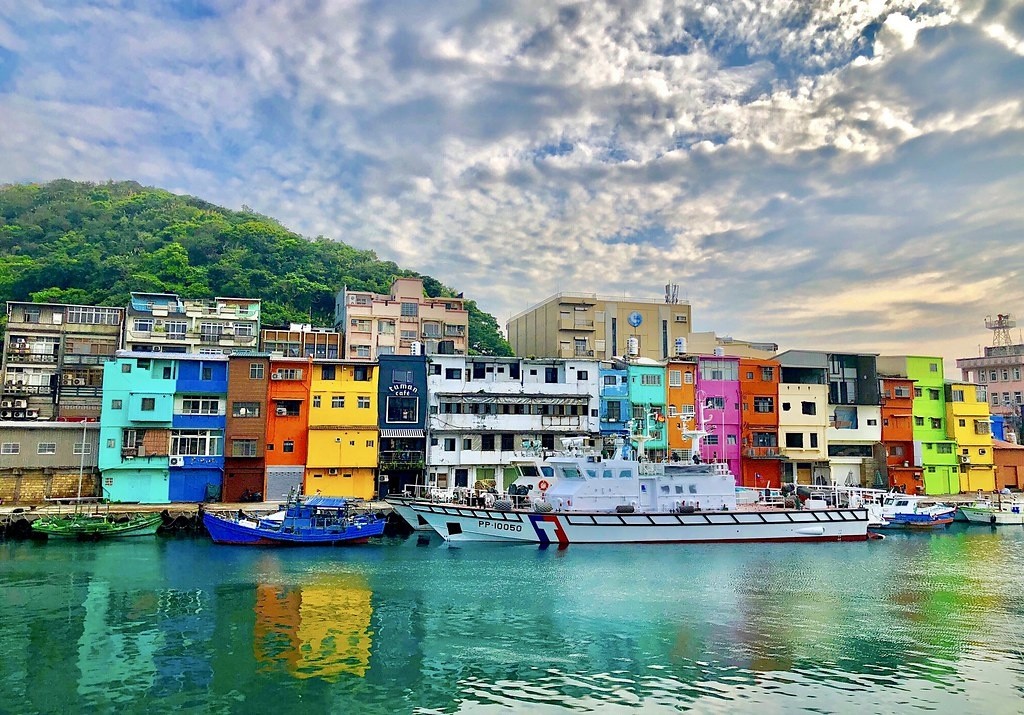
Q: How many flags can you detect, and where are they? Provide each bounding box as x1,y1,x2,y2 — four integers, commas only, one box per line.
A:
656,413,665,422
756,473,763,478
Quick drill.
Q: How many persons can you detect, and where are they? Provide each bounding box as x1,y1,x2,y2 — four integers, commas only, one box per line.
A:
214,511,218,516
570,447,578,458
671,452,681,462
693,451,703,465
601,447,608,459
638,455,648,463
658,456,664,463
239,509,248,519
402,409,413,420
391,444,397,463
1000,485,1012,494
402,444,410,460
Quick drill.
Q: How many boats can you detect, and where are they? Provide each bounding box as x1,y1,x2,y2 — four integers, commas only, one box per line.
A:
384,482,447,532
882,494,958,527
848,494,890,530
952,504,968,522
408,393,884,544
32,416,162,538
202,484,389,546
959,488,1024,526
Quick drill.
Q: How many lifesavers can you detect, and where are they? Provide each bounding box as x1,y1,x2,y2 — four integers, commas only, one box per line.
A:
538,480,548,491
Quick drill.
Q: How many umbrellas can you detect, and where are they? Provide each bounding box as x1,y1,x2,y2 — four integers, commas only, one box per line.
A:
844,470,858,487
873,470,883,488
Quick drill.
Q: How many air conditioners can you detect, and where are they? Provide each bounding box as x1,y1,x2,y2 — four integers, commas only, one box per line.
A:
277,408,287,416
2,401,12,407
271,373,281,380
0,409,12,417
13,412,24,417
26,410,38,418
73,378,84,385
152,346,161,351
225,321,234,328
15,399,27,408
380,475,388,482
346,295,357,305
10,338,29,349
154,319,163,325
62,374,72,385
168,456,184,466
959,456,971,464
328,469,337,476
